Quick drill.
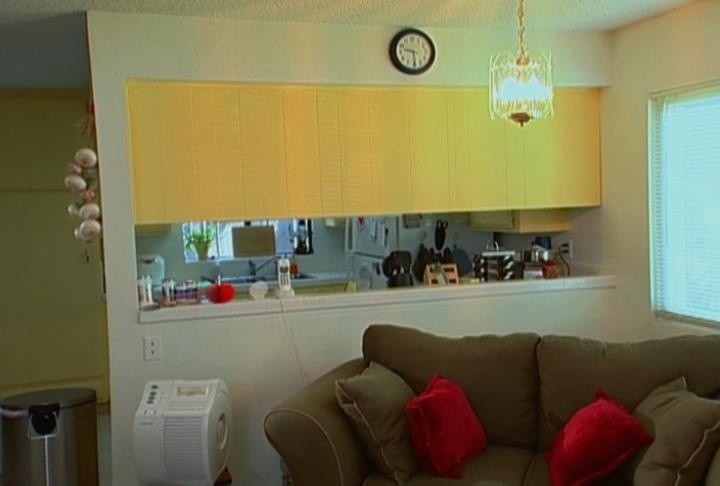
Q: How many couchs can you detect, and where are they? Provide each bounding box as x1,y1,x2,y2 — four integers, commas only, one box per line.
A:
263,324,720,486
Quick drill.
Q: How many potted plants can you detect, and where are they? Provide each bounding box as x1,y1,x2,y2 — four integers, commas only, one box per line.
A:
185,228,217,261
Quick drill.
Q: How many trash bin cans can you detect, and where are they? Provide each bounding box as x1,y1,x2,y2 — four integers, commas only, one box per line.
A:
0,388,100,486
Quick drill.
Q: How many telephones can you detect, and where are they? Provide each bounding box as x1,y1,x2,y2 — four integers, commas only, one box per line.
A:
276,257,295,298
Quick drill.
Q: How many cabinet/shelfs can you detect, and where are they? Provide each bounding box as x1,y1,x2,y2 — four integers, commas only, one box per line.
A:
467,209,574,232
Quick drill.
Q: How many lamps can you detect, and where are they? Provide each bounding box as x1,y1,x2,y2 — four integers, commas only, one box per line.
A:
486,0,554,128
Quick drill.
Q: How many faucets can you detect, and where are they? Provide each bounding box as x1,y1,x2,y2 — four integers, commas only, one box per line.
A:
249,257,278,275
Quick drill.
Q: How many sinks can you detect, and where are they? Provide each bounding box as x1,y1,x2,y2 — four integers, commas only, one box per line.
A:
221,273,314,285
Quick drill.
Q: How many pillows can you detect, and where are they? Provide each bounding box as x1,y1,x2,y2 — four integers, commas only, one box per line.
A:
549,396,648,486
404,377,486,475
334,361,410,484
626,376,720,486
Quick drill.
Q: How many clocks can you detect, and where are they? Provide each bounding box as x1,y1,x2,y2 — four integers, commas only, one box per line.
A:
391,27,436,75
